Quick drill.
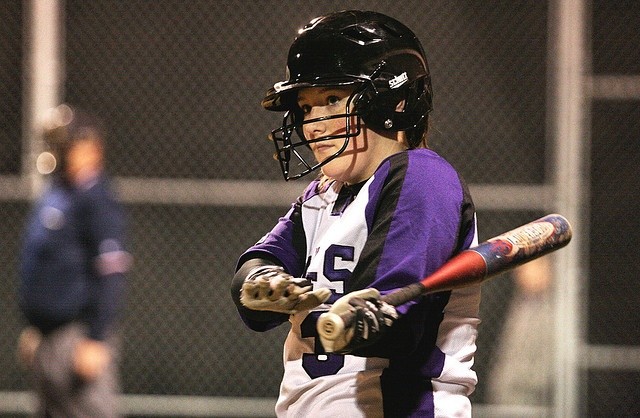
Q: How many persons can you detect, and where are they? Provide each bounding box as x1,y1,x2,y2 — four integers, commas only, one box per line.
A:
229,11,481,418
15,110,130,418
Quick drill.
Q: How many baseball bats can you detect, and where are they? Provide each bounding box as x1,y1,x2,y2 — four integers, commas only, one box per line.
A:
316,213,574,342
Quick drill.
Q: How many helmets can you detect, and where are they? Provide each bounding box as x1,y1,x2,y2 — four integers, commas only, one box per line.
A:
261,10,432,180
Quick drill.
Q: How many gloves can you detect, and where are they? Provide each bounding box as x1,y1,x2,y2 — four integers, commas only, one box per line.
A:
286,288,398,360
240,266,330,315
